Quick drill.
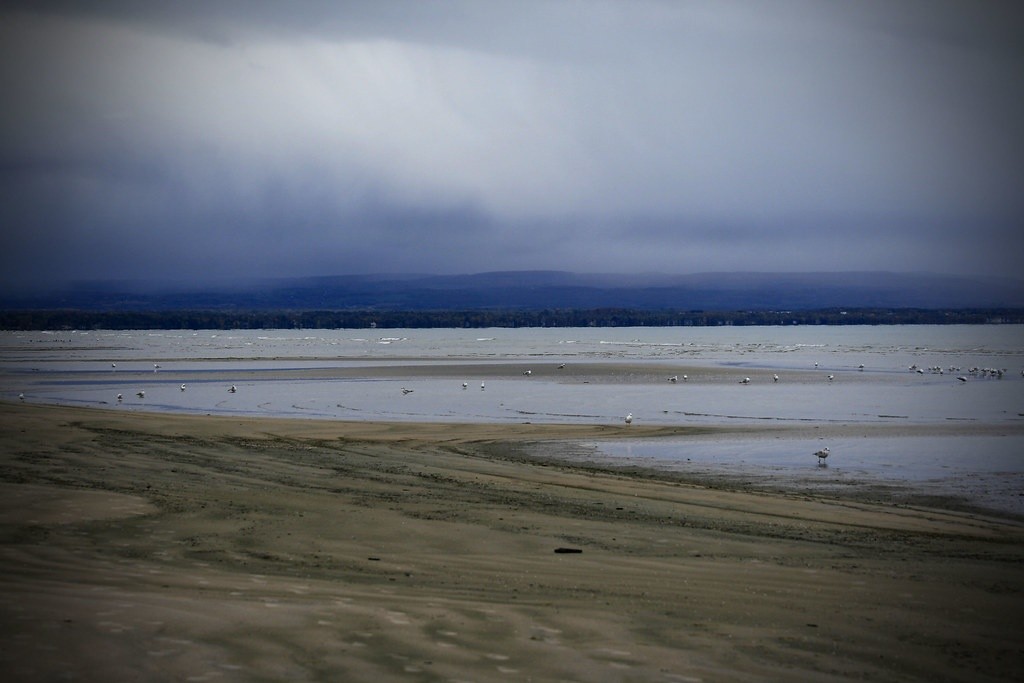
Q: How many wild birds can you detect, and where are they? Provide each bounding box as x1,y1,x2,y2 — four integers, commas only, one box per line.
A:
683,374,688,382
625,413,632,424
739,376,751,385
136,389,145,397
481,381,485,388
812,446,830,465
557,363,566,369
19,392,27,400
117,393,124,399
815,361,818,366
774,374,779,383
522,370,532,376
910,363,1007,384
858,363,864,370
400,386,414,395
181,383,185,391
228,383,237,392
112,363,115,368
828,373,834,381
462,381,468,387
668,375,678,384
